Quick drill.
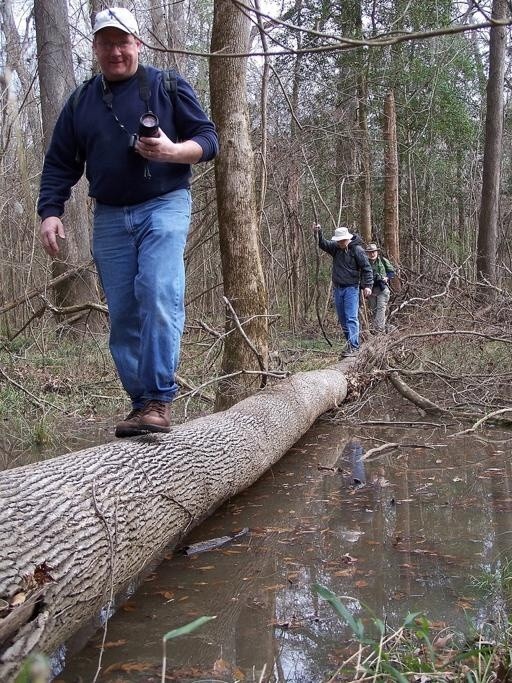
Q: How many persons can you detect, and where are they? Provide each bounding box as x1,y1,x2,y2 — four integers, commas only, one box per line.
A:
38,9,221,438
337,437,387,550
313,223,373,357
365,244,395,334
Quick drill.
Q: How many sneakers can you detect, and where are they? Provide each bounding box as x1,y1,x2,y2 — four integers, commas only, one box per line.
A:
340,346,360,358
137,399,171,433
114,407,152,438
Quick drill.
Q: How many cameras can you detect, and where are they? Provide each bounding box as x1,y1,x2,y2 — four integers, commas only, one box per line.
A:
373,272,382,281
129,111,163,153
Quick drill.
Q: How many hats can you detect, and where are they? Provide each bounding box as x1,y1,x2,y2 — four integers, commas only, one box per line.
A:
92,6,140,39
364,243,380,252
330,226,353,241
